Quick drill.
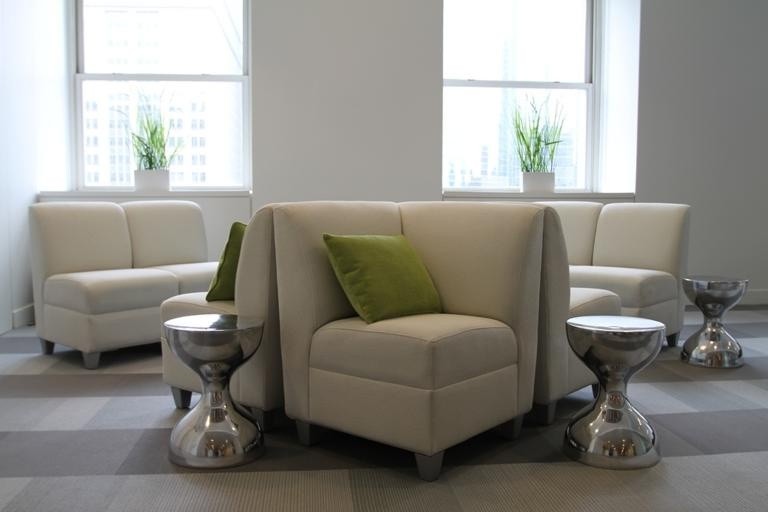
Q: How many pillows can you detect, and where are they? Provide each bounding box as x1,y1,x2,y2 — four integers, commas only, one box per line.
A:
206,222,247,301
322,232,444,322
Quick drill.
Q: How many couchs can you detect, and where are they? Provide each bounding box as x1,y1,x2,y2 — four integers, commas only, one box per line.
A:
157,199,287,439
25,200,220,367
273,199,544,479
533,203,622,426
551,199,690,345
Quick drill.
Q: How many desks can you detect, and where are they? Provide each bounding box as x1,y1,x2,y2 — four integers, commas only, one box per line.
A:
678,274,752,370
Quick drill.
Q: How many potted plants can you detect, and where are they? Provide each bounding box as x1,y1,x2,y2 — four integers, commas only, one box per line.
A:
508,94,566,197
126,106,189,196
